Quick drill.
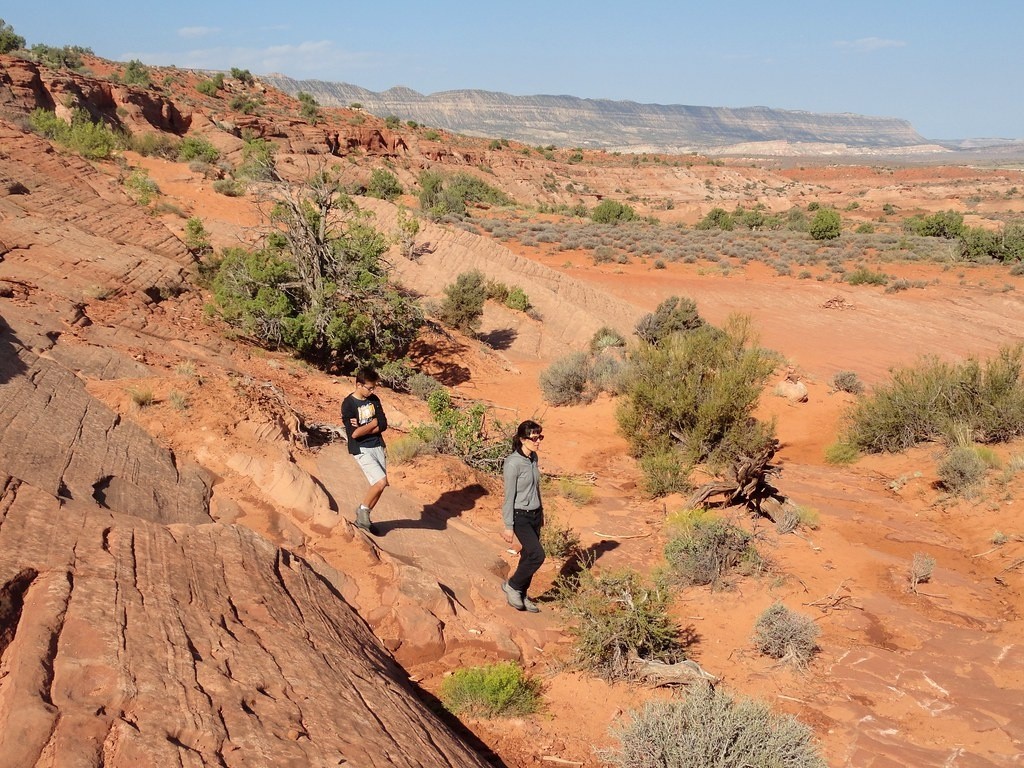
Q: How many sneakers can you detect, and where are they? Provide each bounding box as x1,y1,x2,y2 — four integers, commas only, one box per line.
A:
522,595,539,613
501,582,524,610
354,503,371,532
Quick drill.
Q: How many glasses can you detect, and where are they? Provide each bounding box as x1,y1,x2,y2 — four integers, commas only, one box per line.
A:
522,434,544,442
362,384,376,391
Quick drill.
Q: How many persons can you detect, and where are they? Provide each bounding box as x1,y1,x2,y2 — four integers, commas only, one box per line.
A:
502,420,546,613
341,367,388,534
